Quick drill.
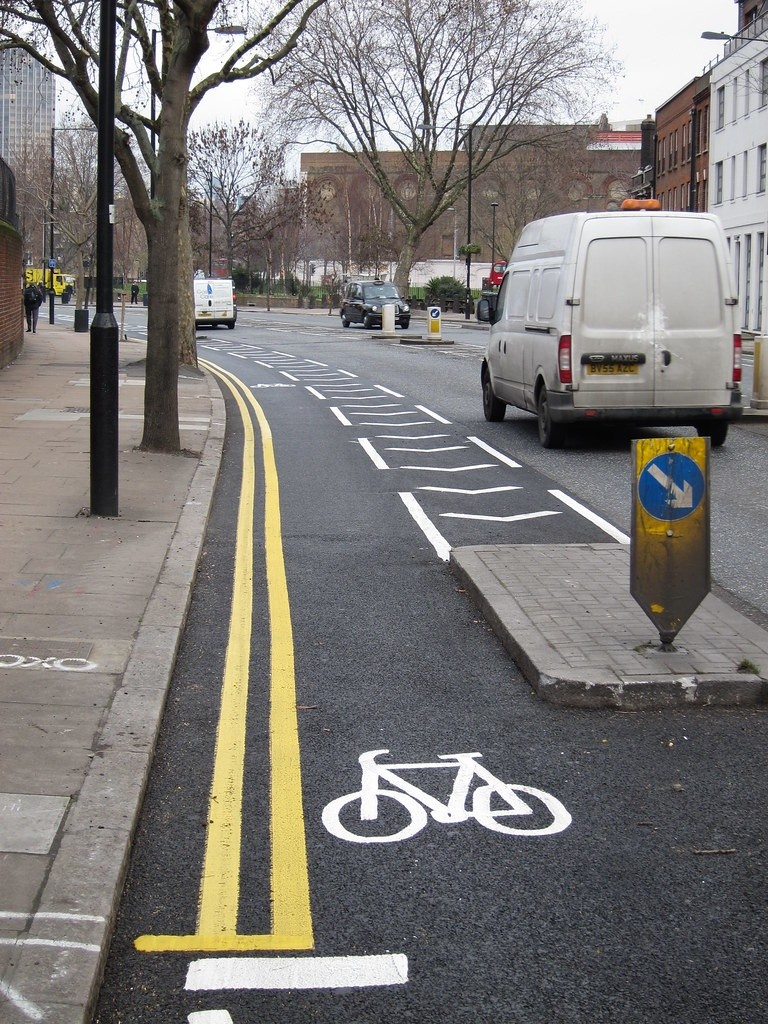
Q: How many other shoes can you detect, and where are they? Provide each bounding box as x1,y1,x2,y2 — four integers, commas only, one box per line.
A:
26,329,31,332
33,330,36,333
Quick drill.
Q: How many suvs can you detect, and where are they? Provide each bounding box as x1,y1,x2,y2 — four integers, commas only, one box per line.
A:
340,279,412,329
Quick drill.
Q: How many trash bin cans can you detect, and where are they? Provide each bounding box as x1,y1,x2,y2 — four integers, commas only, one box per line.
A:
143,294,148,306
481,292,498,310
62,292,69,304
75,310,89,332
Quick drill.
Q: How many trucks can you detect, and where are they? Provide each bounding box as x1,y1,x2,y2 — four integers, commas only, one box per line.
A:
192,276,237,330
24,267,76,296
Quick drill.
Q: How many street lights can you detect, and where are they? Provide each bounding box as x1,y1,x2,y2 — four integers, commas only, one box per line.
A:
490,202,498,263
48,127,98,325
417,123,471,320
204,171,213,277
147,24,246,195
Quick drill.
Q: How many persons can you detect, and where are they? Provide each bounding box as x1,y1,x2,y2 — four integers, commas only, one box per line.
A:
24,283,43,333
131,281,139,304
38,282,46,303
66,284,72,300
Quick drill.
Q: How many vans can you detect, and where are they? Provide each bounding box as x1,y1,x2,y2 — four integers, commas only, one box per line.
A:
480,198,745,451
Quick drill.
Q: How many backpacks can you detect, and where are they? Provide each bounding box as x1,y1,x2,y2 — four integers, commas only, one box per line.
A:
27,286,39,304
